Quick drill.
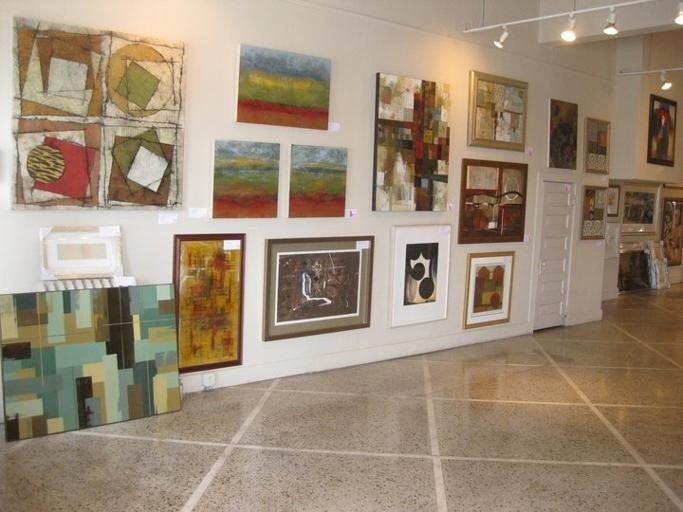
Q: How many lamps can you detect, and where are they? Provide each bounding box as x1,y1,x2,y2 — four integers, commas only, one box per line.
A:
618,67,682,91
673,0,683,26
462,0,683,50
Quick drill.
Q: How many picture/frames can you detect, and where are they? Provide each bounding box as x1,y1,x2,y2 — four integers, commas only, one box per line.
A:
621,182,661,234
647,93,677,166
662,196,683,268
173,234,375,374
458,70,528,330
576,115,620,245
